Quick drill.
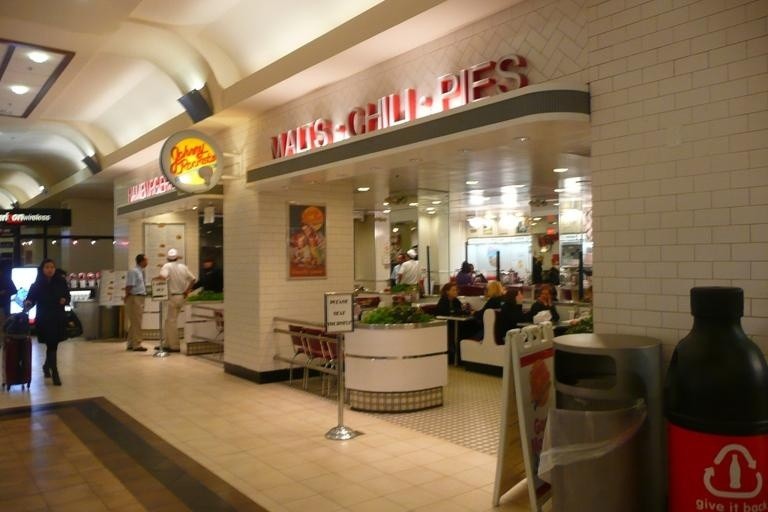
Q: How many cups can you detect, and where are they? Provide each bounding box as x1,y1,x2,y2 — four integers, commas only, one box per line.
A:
569,311,574,320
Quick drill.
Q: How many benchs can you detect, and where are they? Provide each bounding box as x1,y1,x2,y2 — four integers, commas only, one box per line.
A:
357,296,531,377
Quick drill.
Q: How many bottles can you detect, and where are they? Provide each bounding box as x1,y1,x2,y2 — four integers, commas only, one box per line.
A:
661,285,768,512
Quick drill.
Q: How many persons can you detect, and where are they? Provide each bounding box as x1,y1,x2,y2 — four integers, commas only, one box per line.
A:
455,261,473,285
499,288,540,342
390,253,405,285
122,254,149,351
187,258,223,293
0,265,16,348
152,248,195,352
468,263,486,283
396,249,419,286
291,230,326,264
458,279,503,340
23,259,70,386
543,253,559,285
552,286,592,339
530,283,559,321
532,248,544,283
435,282,469,363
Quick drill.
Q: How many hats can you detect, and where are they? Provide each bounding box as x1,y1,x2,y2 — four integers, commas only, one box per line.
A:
408,249,418,258
167,248,179,259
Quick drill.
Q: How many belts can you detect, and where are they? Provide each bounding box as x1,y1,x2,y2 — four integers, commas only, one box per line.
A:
172,294,184,295
136,294,145,296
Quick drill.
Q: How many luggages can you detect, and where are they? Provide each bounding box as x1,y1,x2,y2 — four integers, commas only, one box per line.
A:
1,301,31,393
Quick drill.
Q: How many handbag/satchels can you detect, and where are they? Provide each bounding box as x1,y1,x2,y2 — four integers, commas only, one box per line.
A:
3,303,30,333
59,298,84,338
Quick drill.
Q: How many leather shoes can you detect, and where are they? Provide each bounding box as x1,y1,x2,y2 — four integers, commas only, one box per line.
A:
42,365,50,377
126,343,181,354
53,373,61,385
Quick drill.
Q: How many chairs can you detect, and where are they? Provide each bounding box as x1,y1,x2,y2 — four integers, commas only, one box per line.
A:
288,325,345,397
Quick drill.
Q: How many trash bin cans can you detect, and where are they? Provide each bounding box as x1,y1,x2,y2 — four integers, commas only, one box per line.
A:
538,333,666,512
